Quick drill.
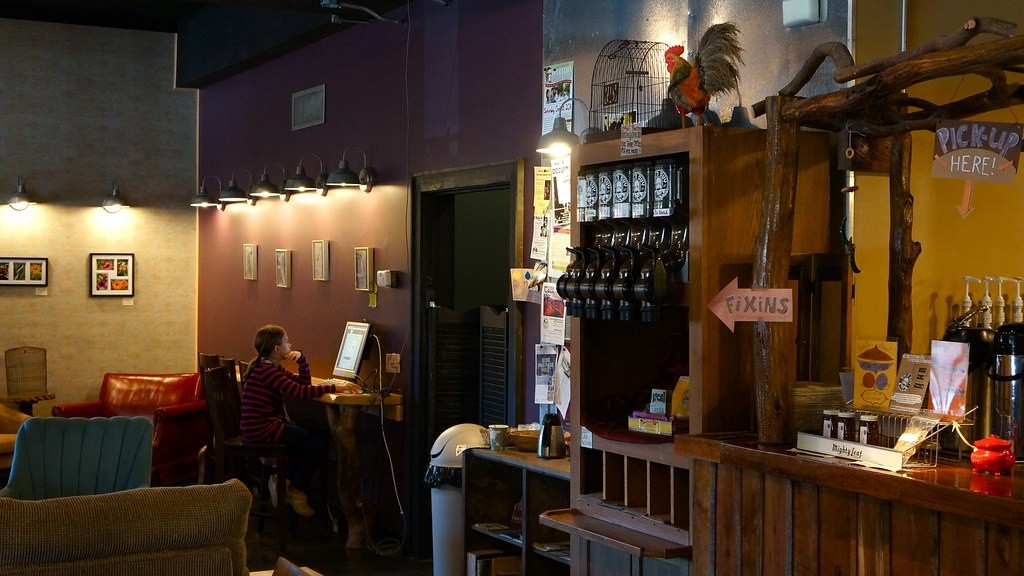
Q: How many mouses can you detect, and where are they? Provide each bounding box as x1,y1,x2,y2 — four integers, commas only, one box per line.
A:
343,388,363,394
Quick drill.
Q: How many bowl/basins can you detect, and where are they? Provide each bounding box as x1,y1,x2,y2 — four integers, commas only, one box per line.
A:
509,430,540,452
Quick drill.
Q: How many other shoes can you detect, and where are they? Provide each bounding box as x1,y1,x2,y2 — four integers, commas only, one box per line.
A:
286,488,313,516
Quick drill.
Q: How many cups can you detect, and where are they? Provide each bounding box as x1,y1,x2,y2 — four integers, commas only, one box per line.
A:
488,424,509,451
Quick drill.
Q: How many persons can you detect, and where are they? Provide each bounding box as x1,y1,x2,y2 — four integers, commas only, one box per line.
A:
239,326,362,517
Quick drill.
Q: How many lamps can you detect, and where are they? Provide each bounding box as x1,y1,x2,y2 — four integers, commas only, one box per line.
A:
189,174,227,211
221,167,259,207
248,159,291,202
320,0,404,24
100,179,130,214
325,145,376,193
7,174,35,210
645,72,763,129
284,151,330,196
532,97,592,157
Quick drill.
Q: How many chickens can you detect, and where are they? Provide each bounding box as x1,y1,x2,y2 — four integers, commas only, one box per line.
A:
664,22,745,128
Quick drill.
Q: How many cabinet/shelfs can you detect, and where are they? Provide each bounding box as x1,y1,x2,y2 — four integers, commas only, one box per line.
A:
461,445,571,576
539,129,834,576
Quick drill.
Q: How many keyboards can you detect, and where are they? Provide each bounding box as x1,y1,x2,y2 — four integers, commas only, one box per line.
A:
314,378,350,386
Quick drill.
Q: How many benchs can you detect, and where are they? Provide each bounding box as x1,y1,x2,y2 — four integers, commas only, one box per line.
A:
0,478,254,576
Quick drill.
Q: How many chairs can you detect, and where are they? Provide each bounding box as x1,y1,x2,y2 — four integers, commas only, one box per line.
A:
0,416,155,501
198,351,300,547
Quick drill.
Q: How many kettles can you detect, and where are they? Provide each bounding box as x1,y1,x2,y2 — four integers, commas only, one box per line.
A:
537,413,565,459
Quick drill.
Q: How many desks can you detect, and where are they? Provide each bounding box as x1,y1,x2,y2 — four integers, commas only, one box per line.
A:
672,427,1024,576
237,362,402,550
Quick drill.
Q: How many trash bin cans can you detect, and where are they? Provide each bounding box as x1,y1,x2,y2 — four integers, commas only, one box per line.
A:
431,423,492,576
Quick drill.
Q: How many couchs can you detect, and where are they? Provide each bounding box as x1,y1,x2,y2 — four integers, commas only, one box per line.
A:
0,404,32,476
51,373,212,490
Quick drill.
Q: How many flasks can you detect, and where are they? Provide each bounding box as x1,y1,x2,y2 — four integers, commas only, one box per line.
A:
940,327,982,452
982,321,1024,464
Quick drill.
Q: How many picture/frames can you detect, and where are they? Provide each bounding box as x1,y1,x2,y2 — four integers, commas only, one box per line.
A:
311,239,329,282
243,243,258,281
90,253,134,297
0,256,48,287
275,249,292,289
353,246,374,292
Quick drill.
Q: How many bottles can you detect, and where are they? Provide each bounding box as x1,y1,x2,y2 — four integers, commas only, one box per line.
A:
857,414,878,445
821,409,840,439
837,412,856,442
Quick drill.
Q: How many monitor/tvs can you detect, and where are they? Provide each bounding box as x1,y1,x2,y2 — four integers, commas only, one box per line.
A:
333,321,375,383
